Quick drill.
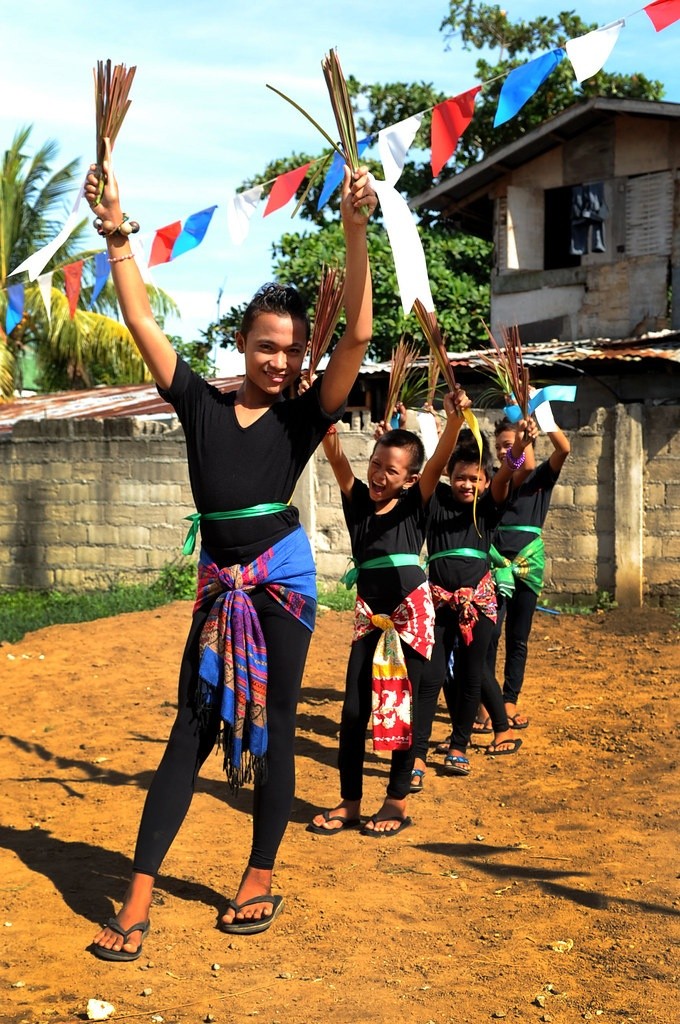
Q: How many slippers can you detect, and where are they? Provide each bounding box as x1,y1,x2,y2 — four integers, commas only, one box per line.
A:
361,811,410,836
436,738,471,754
309,809,361,835
473,714,493,733
444,755,470,775
223,893,284,933
95,914,150,962
506,711,529,729
409,768,425,792
486,738,523,755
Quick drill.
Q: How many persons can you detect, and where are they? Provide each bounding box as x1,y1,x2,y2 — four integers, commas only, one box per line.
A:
83,160,377,960
296,369,570,836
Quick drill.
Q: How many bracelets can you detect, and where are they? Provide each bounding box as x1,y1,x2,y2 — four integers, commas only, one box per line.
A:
324,424,337,434
506,445,525,471
93,213,140,237
107,253,135,262
